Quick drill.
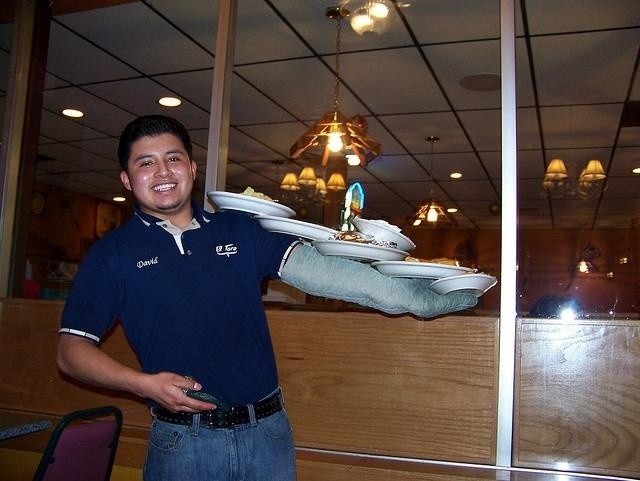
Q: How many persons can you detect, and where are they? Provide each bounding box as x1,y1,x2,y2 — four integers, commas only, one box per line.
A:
55,113,478,480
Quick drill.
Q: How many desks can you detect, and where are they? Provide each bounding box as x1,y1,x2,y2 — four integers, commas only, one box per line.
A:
1,412,52,442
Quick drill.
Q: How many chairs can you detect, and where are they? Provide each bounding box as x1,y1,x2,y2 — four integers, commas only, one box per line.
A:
35,406,123,480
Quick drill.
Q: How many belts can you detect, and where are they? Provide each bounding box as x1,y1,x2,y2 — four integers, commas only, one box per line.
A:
155,391,281,429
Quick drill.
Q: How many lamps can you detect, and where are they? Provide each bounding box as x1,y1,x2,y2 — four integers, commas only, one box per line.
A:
288,6,382,170
573,245,602,277
539,158,606,204
281,166,348,210
335,1,400,38
405,136,458,231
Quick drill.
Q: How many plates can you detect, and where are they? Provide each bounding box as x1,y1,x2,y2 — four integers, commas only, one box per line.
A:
369,259,475,278
352,217,417,255
208,190,296,221
252,214,338,241
428,273,497,299
312,237,412,264
